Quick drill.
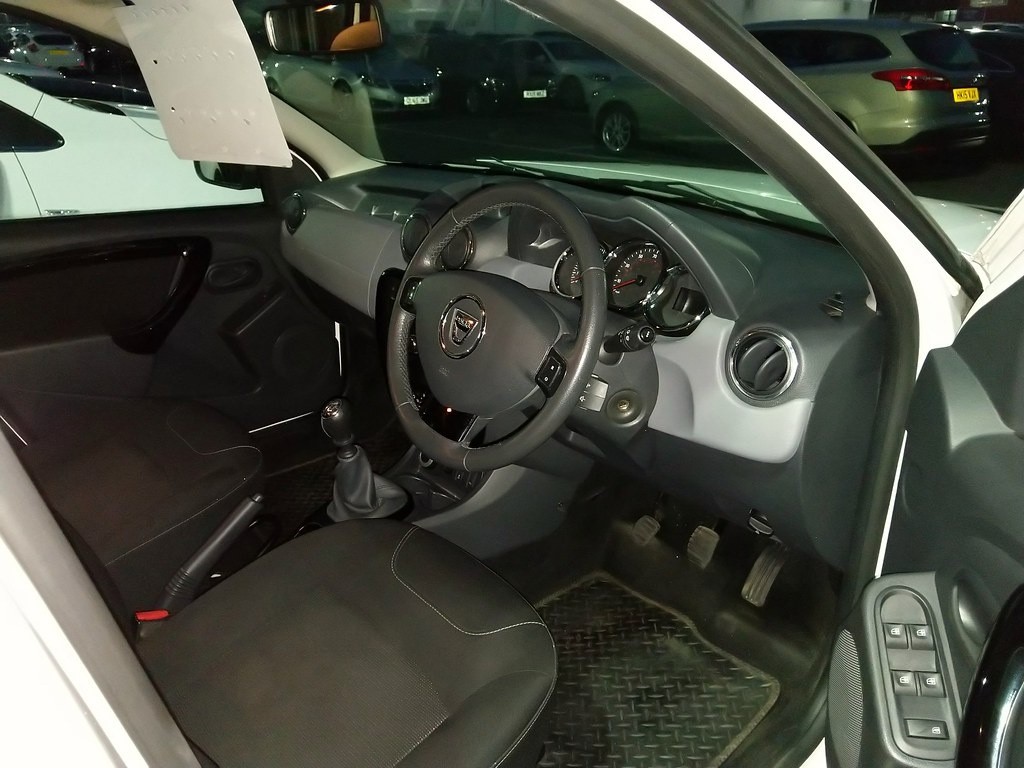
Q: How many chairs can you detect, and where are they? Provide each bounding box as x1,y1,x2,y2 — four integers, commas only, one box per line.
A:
18,398,559,768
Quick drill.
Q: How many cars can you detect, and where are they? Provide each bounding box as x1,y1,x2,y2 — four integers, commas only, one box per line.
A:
0,16,268,218
944,19,1024,144
342,25,623,120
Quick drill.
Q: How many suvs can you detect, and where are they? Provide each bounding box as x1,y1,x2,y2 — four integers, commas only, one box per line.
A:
585,16,992,181
